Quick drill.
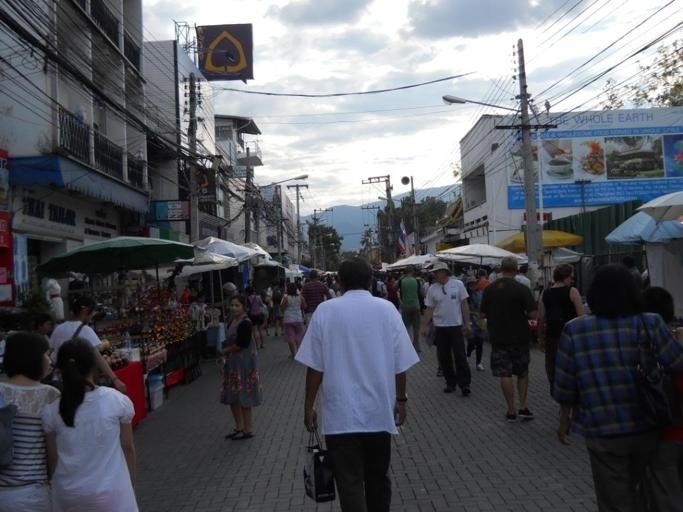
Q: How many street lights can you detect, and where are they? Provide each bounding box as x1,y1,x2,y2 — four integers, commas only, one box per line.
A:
376,195,418,256
440,95,544,258
255,174,307,245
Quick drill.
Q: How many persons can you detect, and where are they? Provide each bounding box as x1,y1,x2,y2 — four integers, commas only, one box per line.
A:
186,270,335,440
1,295,140,512
551,257,682,512
294,259,420,512
372,258,584,421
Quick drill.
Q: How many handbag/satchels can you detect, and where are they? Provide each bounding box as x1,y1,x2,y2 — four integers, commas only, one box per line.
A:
280,295,288,311
303,425,335,504
631,316,675,427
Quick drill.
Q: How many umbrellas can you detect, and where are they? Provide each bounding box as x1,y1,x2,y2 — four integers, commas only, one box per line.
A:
380,228,586,272
633,190,683,225
31,233,273,321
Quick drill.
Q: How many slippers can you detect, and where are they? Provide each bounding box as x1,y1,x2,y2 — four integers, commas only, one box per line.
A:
225,429,242,439
233,431,254,441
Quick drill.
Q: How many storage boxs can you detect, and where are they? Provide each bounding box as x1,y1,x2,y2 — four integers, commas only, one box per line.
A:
145,384,164,410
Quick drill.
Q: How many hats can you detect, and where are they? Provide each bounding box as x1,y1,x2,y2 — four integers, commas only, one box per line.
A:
423,263,433,270
428,261,450,272
465,275,479,284
222,282,237,291
501,256,517,268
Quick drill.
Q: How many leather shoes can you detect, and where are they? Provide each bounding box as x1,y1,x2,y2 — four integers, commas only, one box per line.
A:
461,386,470,396
444,385,455,394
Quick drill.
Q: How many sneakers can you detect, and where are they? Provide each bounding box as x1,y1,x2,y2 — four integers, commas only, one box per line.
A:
504,411,517,423
518,407,534,419
476,364,484,371
